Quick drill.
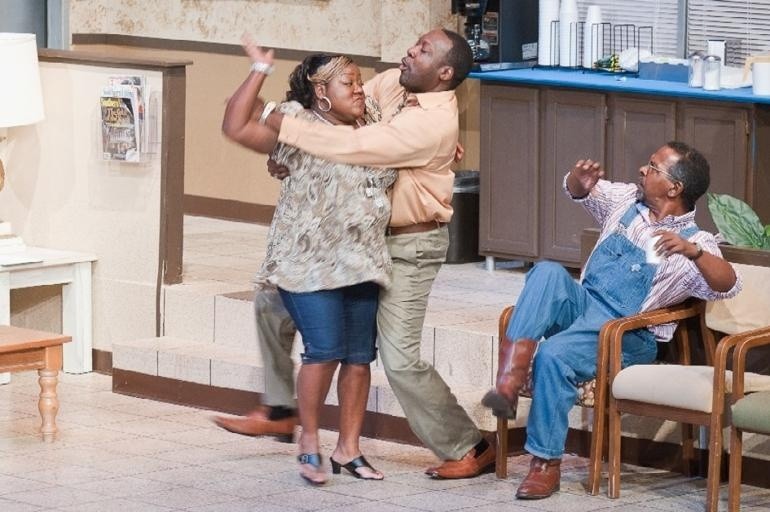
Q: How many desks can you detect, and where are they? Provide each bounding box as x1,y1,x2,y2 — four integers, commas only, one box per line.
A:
0,324,73,445
0,246,97,383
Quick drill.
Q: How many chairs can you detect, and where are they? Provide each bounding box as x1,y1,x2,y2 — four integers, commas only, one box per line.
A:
728,336,770,512
494,295,716,495
608,268,769,511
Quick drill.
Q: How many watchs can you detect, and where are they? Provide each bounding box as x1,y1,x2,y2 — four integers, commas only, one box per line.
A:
688,241,704,261
251,63,276,76
258,101,277,126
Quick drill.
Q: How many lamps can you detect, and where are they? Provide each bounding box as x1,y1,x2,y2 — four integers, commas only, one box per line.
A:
0,31,46,258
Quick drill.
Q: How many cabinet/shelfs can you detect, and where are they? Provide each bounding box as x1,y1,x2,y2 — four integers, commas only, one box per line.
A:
606,88,755,274
478,82,609,282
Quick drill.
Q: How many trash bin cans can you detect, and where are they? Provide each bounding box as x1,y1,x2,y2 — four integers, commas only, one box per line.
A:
444,169,486,265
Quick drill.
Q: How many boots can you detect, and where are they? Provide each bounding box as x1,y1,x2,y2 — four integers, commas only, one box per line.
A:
515,460,562,500
480,339,537,420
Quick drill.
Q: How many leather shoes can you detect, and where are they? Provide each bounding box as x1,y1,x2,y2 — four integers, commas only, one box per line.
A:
210,407,298,443
424,445,496,480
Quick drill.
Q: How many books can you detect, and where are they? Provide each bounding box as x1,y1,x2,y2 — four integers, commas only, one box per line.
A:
99,73,148,161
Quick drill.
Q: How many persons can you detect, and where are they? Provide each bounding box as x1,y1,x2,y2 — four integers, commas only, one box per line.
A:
481,142,742,498
210,28,498,480
223,38,463,489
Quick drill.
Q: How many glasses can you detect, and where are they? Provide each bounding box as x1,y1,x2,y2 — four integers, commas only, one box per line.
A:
646,161,679,186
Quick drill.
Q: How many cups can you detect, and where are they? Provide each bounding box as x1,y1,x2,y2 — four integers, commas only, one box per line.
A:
751,62,770,96
583,4,604,70
537,0,561,67
561,0,582,68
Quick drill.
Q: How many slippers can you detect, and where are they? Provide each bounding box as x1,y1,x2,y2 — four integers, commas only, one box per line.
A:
298,454,328,486
330,454,383,482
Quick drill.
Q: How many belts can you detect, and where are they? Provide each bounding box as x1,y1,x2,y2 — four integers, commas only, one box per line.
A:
386,220,441,235
